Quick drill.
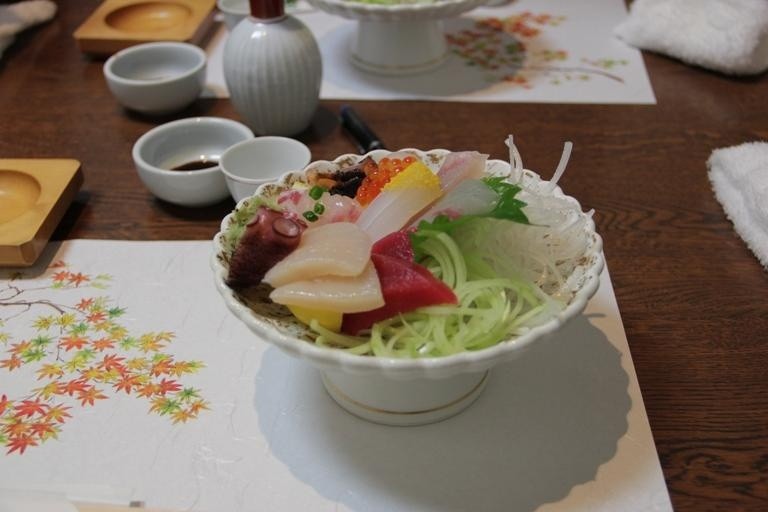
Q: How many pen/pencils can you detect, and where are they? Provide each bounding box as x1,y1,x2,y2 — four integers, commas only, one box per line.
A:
338,105,387,154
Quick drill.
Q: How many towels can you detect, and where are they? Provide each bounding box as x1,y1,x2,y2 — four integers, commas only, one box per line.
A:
612,1,768,76
703,140,768,274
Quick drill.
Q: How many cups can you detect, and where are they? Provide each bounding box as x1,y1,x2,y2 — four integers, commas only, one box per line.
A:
219,136,312,203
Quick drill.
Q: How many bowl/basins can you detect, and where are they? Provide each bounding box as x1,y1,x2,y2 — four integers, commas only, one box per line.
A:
102,42,208,113
216,0,248,30
131,116,256,207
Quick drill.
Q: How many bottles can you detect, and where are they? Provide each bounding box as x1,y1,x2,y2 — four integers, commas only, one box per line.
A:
222,0,323,134
